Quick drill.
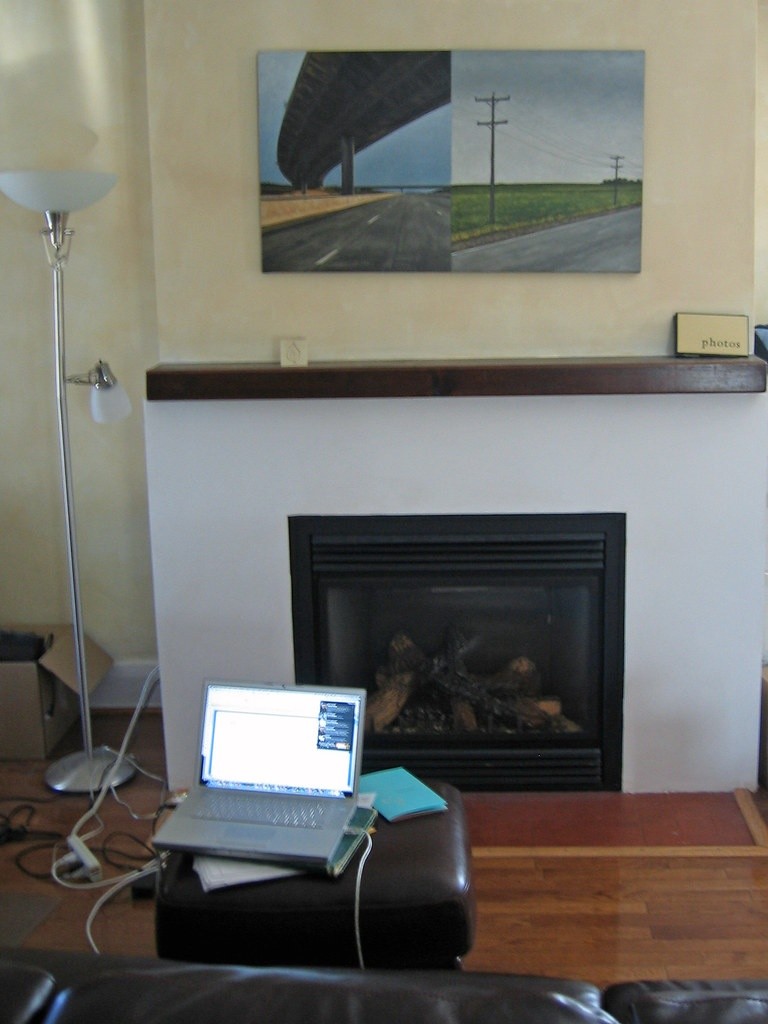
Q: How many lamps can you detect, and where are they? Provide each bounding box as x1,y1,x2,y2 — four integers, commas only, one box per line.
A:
0,169,132,792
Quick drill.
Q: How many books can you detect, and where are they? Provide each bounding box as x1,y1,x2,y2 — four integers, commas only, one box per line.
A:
272,807,378,877
358,764,449,822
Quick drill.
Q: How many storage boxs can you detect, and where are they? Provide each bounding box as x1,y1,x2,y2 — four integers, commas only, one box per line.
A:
0,623,114,762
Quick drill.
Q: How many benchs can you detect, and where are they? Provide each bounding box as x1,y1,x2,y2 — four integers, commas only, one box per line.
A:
0,946,768,1024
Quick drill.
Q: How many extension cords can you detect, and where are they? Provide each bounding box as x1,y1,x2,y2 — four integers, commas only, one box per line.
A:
63,834,103,883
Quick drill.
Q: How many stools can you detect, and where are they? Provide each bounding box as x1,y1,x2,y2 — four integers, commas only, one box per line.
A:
154,780,474,972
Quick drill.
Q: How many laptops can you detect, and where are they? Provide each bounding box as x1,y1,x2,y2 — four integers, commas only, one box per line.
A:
151,679,367,863
